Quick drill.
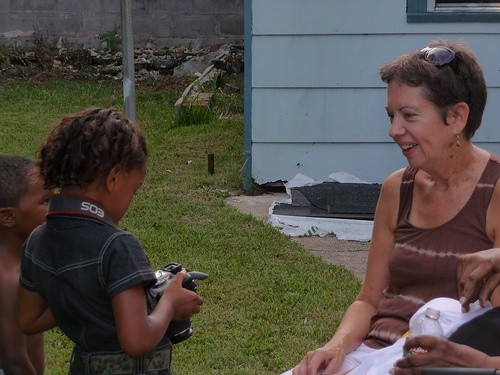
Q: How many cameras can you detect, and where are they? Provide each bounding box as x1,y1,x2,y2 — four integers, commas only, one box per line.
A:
147,262,209,345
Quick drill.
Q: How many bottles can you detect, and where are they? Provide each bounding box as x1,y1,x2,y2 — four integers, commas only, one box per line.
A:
402,307,444,357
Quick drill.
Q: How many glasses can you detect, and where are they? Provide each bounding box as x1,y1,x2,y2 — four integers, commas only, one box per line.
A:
417,46,473,102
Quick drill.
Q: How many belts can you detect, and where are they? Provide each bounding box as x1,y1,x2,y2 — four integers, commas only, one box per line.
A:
70,347,171,375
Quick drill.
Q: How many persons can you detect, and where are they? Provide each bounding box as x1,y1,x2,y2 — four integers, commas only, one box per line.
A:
16,107,204,375
0,154,56,375
280,41,500,375
